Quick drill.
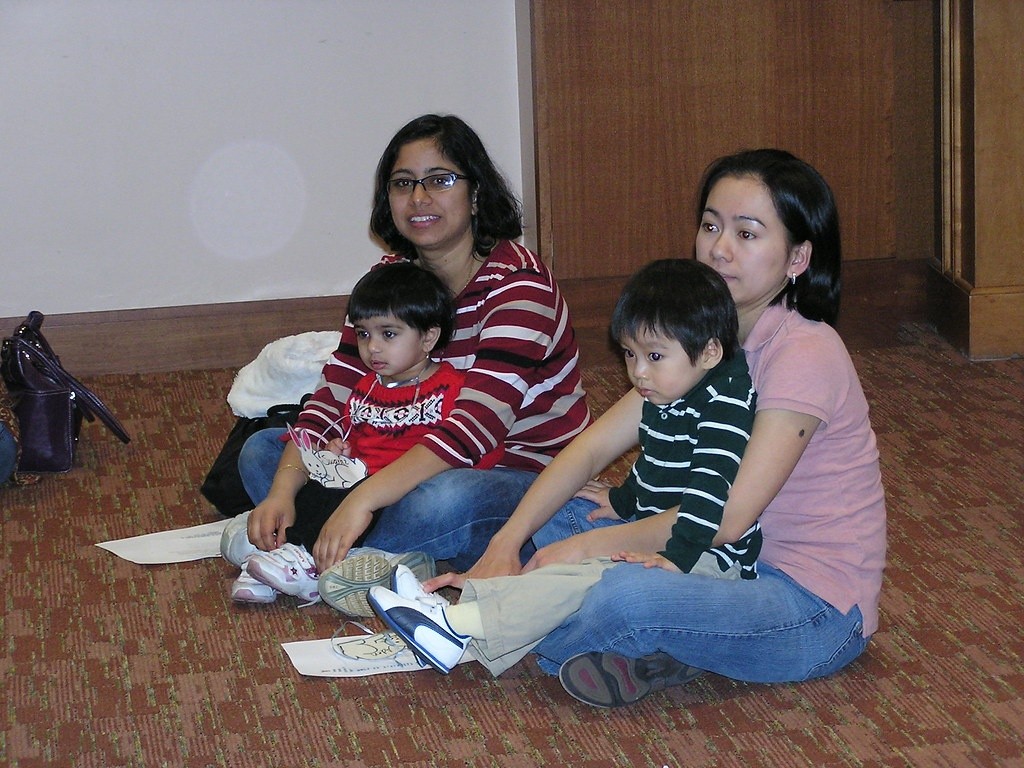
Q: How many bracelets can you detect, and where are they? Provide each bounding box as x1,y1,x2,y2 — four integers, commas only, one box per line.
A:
274,465,310,484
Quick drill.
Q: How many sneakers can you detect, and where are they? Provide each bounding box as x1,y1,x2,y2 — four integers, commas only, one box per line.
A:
318,546,437,617
389,563,451,668
366,586,473,675
220,509,278,567
231,562,277,604
246,544,323,608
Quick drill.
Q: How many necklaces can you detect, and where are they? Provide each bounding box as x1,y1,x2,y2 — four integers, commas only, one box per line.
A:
386,361,432,389
456,254,474,296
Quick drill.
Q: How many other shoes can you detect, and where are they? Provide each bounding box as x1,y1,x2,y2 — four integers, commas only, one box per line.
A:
559,649,705,708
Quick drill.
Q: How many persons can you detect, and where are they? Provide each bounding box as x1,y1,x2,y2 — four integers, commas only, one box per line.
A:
366,257,767,676
424,147,888,709
232,263,504,605
220,113,593,618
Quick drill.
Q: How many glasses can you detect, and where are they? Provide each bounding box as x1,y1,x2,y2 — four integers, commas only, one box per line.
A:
386,172,468,196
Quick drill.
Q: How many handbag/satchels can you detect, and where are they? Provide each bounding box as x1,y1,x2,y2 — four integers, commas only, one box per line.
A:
199,393,313,518
0,310,131,475
